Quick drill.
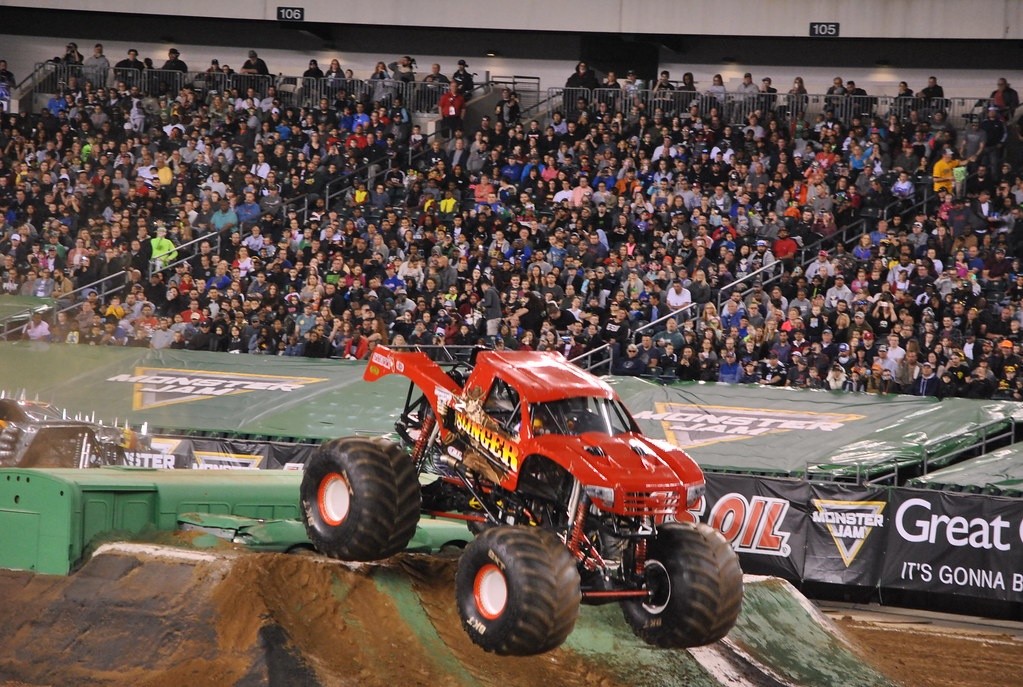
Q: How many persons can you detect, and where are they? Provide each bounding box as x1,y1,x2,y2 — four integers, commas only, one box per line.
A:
533,398,575,434
0,42,1022,406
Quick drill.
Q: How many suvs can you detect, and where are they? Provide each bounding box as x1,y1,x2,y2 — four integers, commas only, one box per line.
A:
300,339,744,658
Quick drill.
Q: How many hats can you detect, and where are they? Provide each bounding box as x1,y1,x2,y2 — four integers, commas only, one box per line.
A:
3,103,1012,351
